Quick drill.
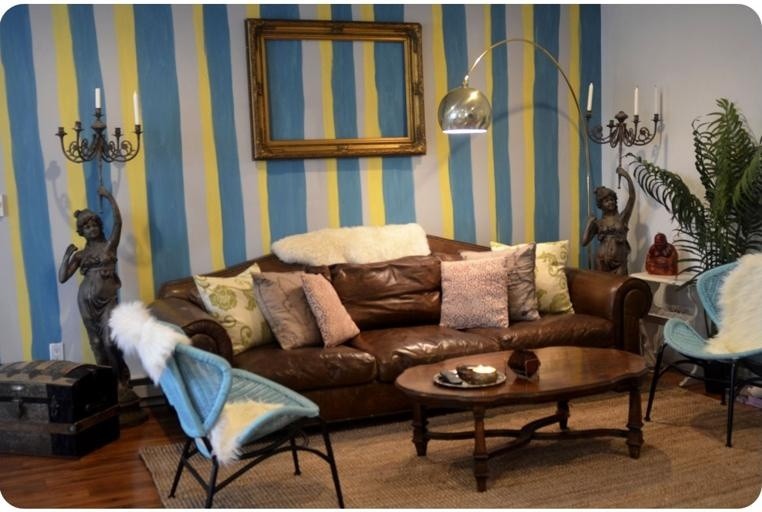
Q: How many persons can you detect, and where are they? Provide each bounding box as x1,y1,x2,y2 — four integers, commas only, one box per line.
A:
580,165,637,274
55,185,126,370
645,232,679,277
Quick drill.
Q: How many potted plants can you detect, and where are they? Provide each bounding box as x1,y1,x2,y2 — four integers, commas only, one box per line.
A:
622,95,762,393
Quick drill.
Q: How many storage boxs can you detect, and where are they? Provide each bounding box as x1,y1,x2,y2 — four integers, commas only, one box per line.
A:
0,359,120,460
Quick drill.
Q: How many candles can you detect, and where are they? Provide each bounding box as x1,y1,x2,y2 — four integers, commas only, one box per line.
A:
95,87,101,109
587,83,593,111
634,88,638,115
132,91,140,126
654,86,662,114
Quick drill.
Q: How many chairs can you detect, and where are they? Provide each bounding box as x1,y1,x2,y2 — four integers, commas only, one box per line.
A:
109,300,344,509
644,252,761,447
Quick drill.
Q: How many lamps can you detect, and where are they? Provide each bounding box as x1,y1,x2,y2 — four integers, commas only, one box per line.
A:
438,38,590,270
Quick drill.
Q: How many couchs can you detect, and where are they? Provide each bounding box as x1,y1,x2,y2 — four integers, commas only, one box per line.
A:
146,222,652,430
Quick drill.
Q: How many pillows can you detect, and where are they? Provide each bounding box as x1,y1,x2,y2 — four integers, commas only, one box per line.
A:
247,271,323,351
299,274,360,349
490,240,575,314
456,243,541,321
193,262,272,356
438,256,510,330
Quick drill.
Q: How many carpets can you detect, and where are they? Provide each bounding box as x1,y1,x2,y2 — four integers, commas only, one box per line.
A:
137,383,761,508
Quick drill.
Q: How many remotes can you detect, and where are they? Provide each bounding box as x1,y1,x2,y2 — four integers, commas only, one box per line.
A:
440,370,462,384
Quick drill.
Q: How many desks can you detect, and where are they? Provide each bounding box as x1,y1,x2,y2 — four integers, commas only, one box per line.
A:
630,271,701,386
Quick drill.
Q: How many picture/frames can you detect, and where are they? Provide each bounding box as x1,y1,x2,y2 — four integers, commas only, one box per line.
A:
245,18,426,160
